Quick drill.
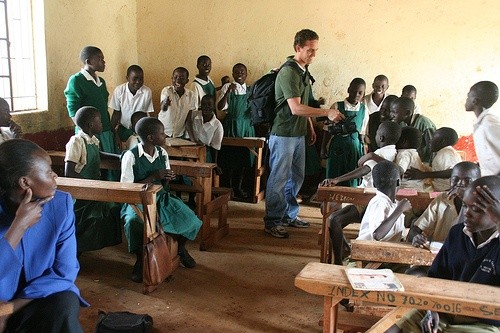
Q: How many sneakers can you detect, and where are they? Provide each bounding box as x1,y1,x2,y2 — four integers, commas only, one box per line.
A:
178,249,196,269
264,225,289,238
280,216,310,228
131,258,143,283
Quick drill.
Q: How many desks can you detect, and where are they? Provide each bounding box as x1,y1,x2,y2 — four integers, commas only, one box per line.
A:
46,137,468,332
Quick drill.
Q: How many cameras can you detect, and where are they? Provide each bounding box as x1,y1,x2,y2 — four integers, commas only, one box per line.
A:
327,121,358,136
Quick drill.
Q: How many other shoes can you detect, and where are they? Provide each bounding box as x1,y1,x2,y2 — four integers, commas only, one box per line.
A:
232,187,249,200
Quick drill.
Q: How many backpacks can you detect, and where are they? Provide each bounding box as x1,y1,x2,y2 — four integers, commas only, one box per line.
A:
94,310,153,333
247,62,303,126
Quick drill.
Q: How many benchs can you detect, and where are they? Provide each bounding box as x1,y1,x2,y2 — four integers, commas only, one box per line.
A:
318,223,359,261
319,310,382,333
160,183,232,218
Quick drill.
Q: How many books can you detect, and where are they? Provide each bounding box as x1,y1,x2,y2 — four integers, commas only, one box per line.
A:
345,267,403,292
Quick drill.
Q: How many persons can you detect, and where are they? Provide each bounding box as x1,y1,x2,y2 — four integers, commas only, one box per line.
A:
405,160,481,276
0,139,92,333
156,67,197,198
0,96,23,145
339,160,412,307
319,120,401,267
263,28,347,238
119,117,204,282
64,106,124,252
361,75,469,230
191,55,230,116
184,94,223,210
217,63,257,198
63,46,116,180
383,174,500,333
464,81,500,177
107,62,155,142
320,78,370,187
